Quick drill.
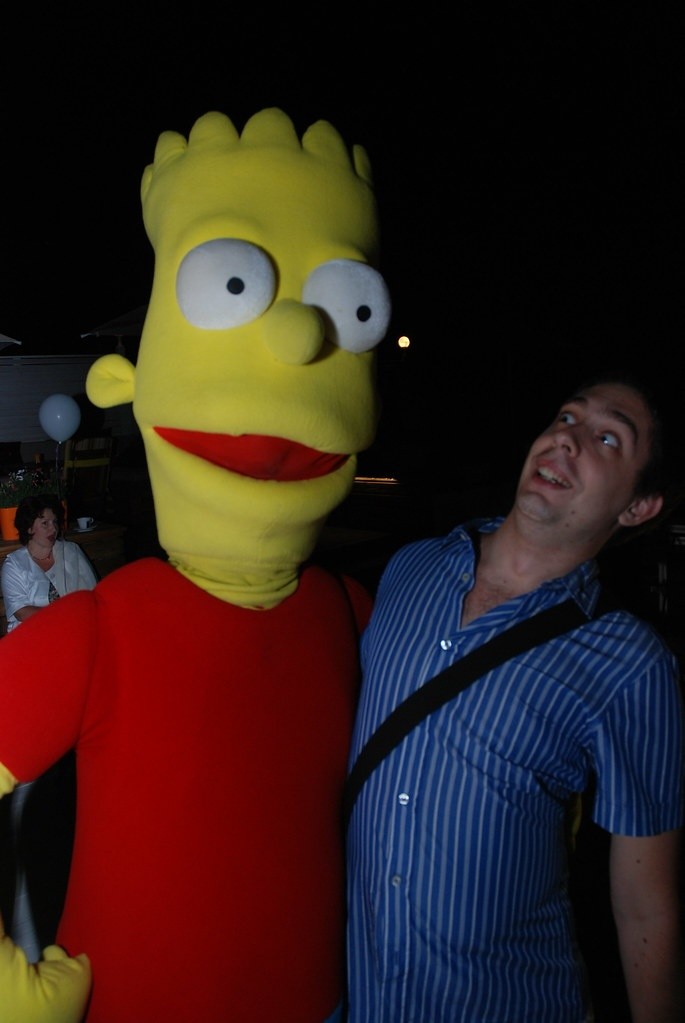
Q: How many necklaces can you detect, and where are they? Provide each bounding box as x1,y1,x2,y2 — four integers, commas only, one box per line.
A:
27,547,52,560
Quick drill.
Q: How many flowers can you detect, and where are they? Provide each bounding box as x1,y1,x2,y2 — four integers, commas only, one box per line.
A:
0,463,69,508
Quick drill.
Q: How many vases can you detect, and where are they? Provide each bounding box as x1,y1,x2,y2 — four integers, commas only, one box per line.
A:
0,498,67,541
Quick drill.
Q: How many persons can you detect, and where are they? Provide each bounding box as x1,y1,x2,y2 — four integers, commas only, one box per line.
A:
335,357,685,1023
0,492,101,634
0,106,388,1023
